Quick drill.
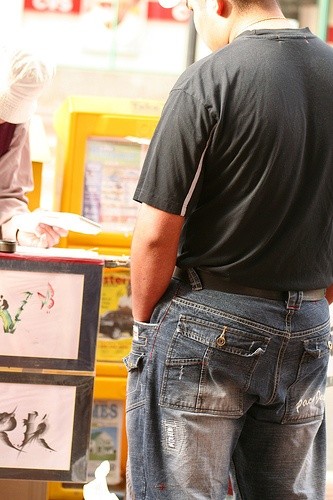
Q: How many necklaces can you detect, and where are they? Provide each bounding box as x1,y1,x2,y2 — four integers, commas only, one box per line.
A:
233,15,288,42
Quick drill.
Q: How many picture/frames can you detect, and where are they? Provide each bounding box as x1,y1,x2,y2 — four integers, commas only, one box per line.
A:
1,372,98,486
0,256,103,375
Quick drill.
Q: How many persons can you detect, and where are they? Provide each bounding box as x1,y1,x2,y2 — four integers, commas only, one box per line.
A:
0,50,69,249
128,0,333,500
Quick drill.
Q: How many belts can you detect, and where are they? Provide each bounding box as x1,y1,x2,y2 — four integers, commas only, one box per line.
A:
171,265,327,303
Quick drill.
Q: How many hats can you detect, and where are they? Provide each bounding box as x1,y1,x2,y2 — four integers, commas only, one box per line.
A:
0,58,42,125
